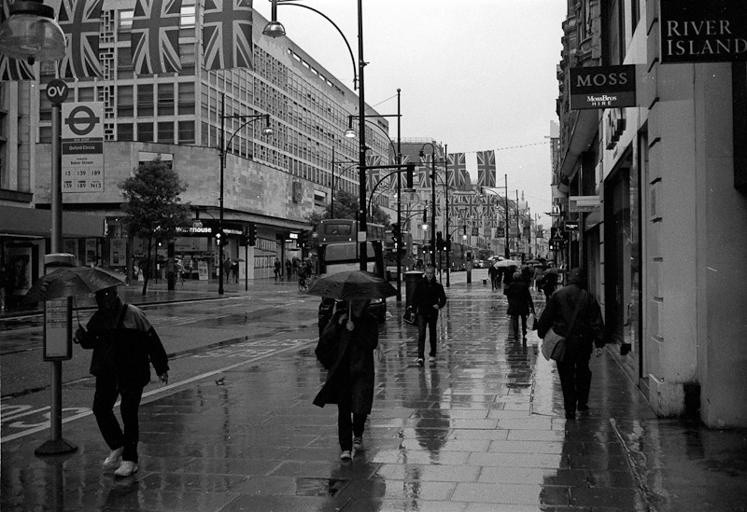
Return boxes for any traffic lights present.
[214,230,222,246]
[248,224,258,246]
[222,232,229,246]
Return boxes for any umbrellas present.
[233,258,246,263]
[24,267,127,344]
[306,271,399,330]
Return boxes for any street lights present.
[259,0,371,275]
[413,140,438,289]
[478,174,540,265]
[446,225,468,289]
[1,0,67,66]
[312,145,369,239]
[343,85,428,302]
[193,91,273,297]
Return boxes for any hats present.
[569,267,585,281]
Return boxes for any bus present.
[315,217,496,272]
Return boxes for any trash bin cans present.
[404,271,424,310]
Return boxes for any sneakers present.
[340,436,362,460]
[103,448,139,477]
[417,357,436,367]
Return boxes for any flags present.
[196,0,255,71]
[55,0,103,77]
[2,50,35,82]
[130,0,184,76]
[356,150,509,229]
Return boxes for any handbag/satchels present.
[541,326,567,362]
[402,308,418,326]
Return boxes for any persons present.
[406,265,447,368]
[77,286,170,477]
[316,298,379,460]
[506,273,535,339]
[537,268,606,419]
[223,258,232,283]
[232,261,240,284]
[273,255,315,289]
[488,255,567,293]
